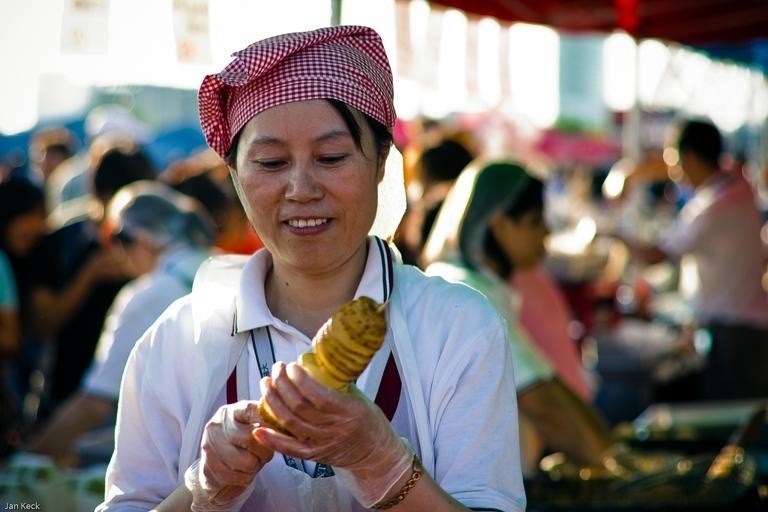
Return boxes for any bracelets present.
[367,454,422,510]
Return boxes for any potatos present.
[256,294,386,438]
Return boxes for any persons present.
[0,116,768,468]
[90,23,527,512]
